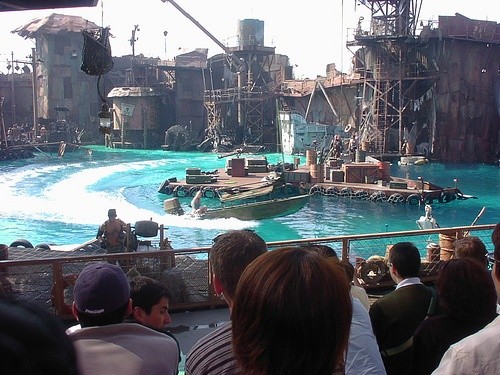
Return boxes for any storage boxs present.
[231,157,267,177]
[341,163,380,184]
[185,168,214,184]
[330,170,343,181]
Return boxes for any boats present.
[349,258,443,294]
[158,170,462,207]
[0,243,227,321]
[164,194,313,223]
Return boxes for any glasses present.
[485,252,500,264]
[212,229,256,247]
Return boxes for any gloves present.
[101,242,106,250]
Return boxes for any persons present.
[124,275,182,365]
[349,134,358,162]
[417,206,437,231]
[401,140,413,157]
[219,245,353,375]
[413,258,491,375]
[96,208,130,271]
[452,237,493,276]
[330,134,343,157]
[369,243,443,375]
[184,229,283,375]
[6,122,48,144]
[430,223,499,375]
[340,260,370,310]
[301,244,385,375]
[0,292,78,374]
[63,261,182,375]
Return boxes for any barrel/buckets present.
[439,231,469,260]
[310,164,324,183]
[356,151,365,162]
[426,244,440,261]
[362,142,369,153]
[306,150,317,166]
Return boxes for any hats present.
[72,264,131,315]
[107,209,117,216]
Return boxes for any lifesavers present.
[281,183,297,193]
[173,186,217,198]
[406,192,433,205]
[439,191,454,203]
